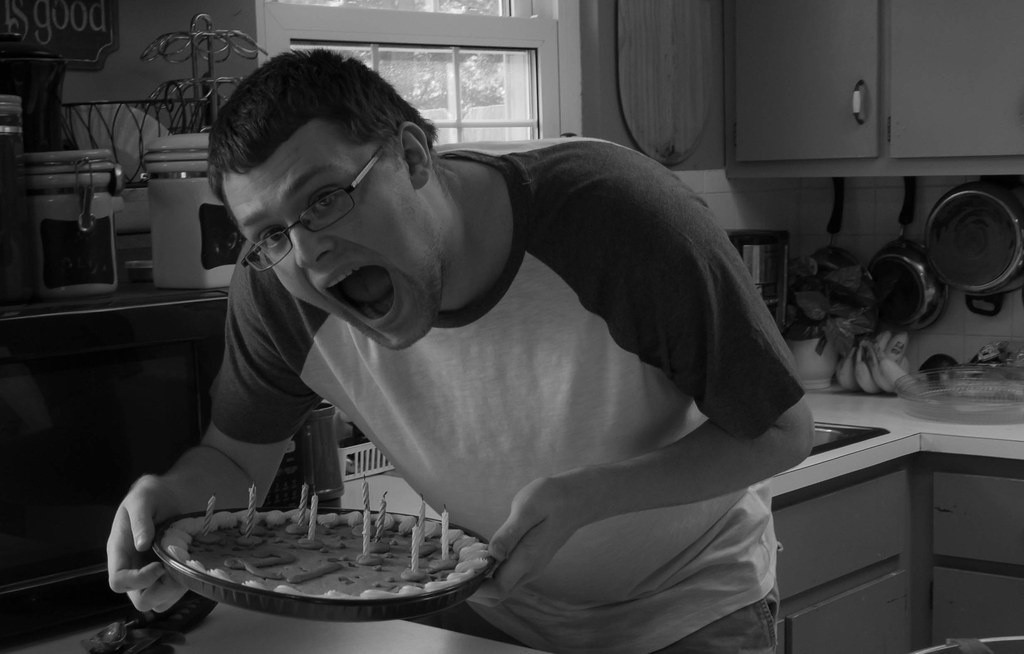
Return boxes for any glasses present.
[240,137,391,272]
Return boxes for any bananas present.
[835,329,913,393]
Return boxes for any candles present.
[411,516,422,575]
[301,479,309,527]
[308,484,317,539]
[363,504,372,556]
[362,474,370,512]
[442,504,449,561]
[374,490,389,543]
[244,481,258,538]
[201,487,218,537]
[419,492,426,547]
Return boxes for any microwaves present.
[0,290,229,642]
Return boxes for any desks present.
[0,602,551,654]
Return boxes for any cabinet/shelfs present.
[770,464,919,654]
[922,467,1024,649]
[721,0,1024,179]
[0,286,342,649]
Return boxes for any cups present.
[295,403,346,502]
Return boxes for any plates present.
[67,100,171,182]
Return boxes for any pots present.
[925,175,1024,316]
[801,176,853,285]
[866,177,948,332]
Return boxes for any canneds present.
[19,148,118,300]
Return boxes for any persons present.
[107,49,815,654]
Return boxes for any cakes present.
[158,509,491,599]
